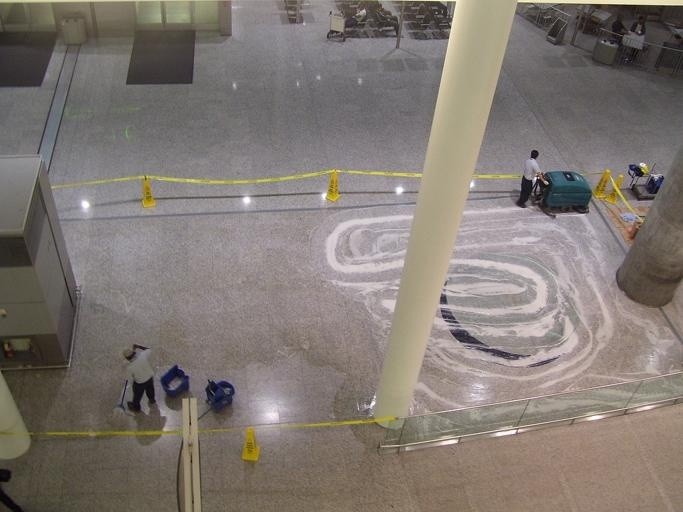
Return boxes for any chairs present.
[283,0,455,39]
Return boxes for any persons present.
[374,7,398,36]
[629,16,646,57]
[121,343,156,413]
[515,149,548,208]
[611,14,628,47]
[344,3,366,28]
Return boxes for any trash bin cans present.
[60,16,87,45]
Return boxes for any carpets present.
[126,30,195,84]
[0,30,59,88]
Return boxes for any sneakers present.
[127,402,140,412]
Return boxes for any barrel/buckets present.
[160,364,189,397]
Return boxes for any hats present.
[123,349,133,357]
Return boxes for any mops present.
[113,345,136,416]
